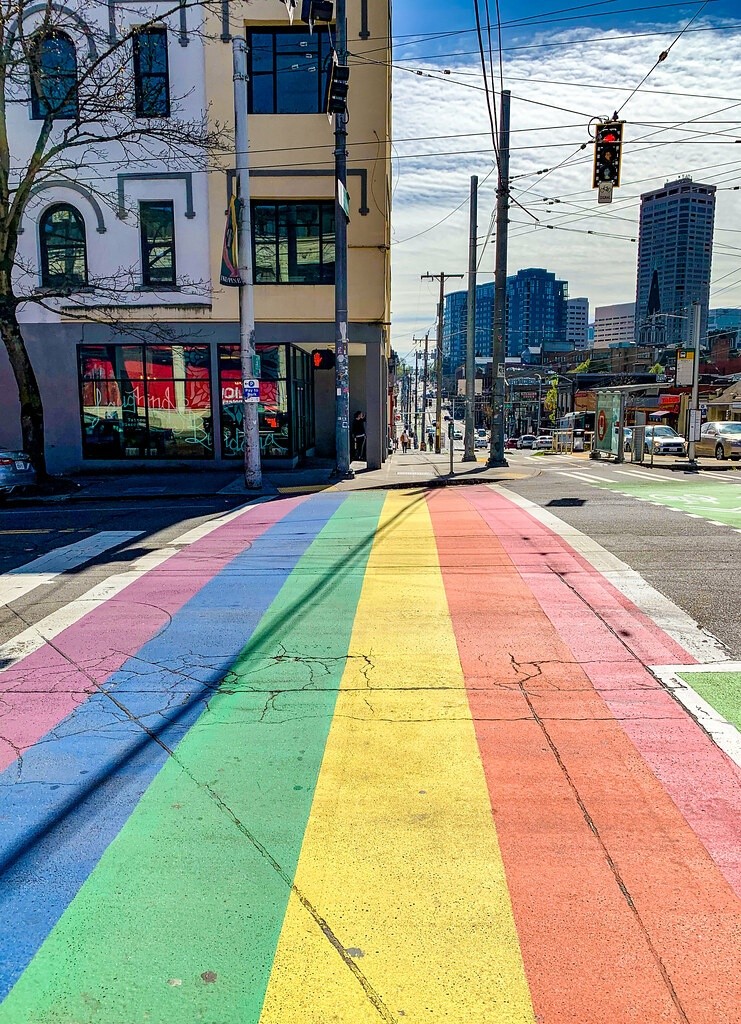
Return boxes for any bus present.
[552,410,597,451]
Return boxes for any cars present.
[453,430,463,440]
[426,420,436,437]
[444,415,454,421]
[685,420,741,460]
[475,439,487,448]
[505,438,519,450]
[83,412,117,454]
[625,424,687,456]
[0,445,39,508]
[591,425,632,450]
[105,417,176,448]
[531,436,553,451]
[478,429,487,437]
[202,402,290,437]
[516,434,537,450]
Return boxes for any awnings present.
[649,411,671,418]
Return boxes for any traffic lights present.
[302,0,332,34]
[593,121,623,187]
[324,49,350,122]
[311,348,335,370]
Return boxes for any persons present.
[401,429,409,453]
[428,434,434,452]
[352,410,367,461]
